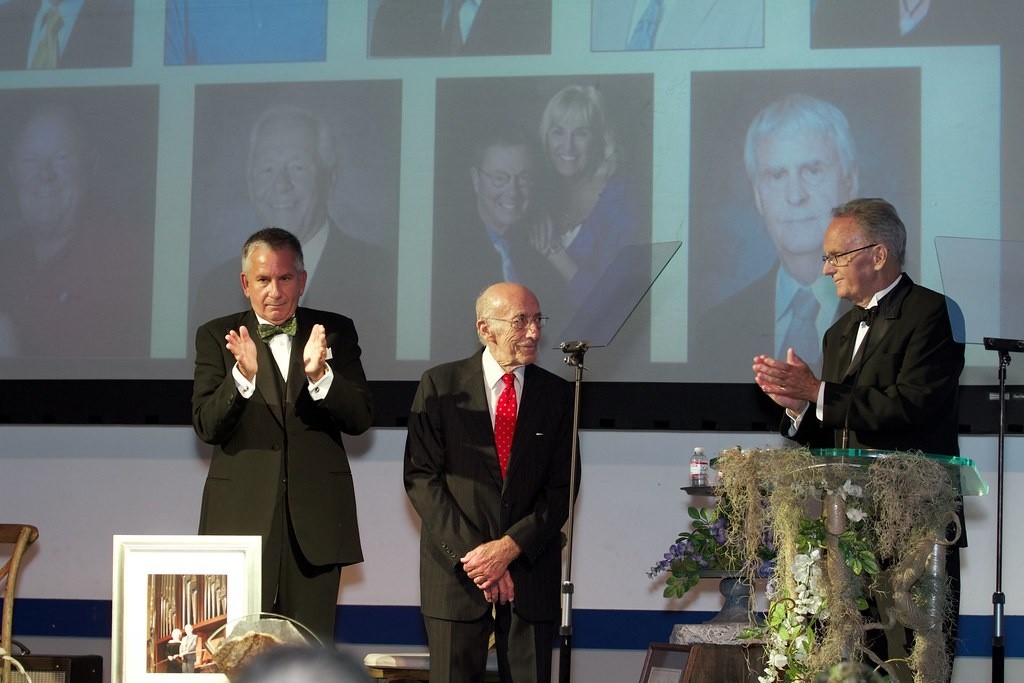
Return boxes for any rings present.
[779,380,787,387]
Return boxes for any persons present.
[812,0,997,48]
[0,0,133,71]
[370,0,552,54]
[167,629,182,672]
[699,95,857,384]
[0,98,150,358]
[440,84,646,355]
[591,1,763,49]
[180,624,198,672]
[230,645,374,682]
[164,0,327,64]
[403,282,581,683]
[191,226,374,646]
[197,109,385,366]
[752,199,967,682]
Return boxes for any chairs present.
[0,523,41,683]
[364,632,497,683]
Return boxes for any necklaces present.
[899,1,922,34]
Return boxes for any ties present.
[493,373,517,481]
[495,236,518,283]
[776,288,819,376]
[628,0,666,52]
[28,7,65,72]
[440,0,469,59]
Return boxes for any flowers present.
[646,504,779,600]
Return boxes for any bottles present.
[690,446,709,487]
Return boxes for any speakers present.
[0,655,103,683]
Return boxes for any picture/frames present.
[111,534,264,683]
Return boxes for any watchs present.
[546,242,563,259]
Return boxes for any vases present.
[695,569,771,622]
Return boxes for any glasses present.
[483,313,549,330]
[821,243,890,266]
[476,165,538,189]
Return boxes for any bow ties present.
[850,304,880,326]
[256,317,298,344]
